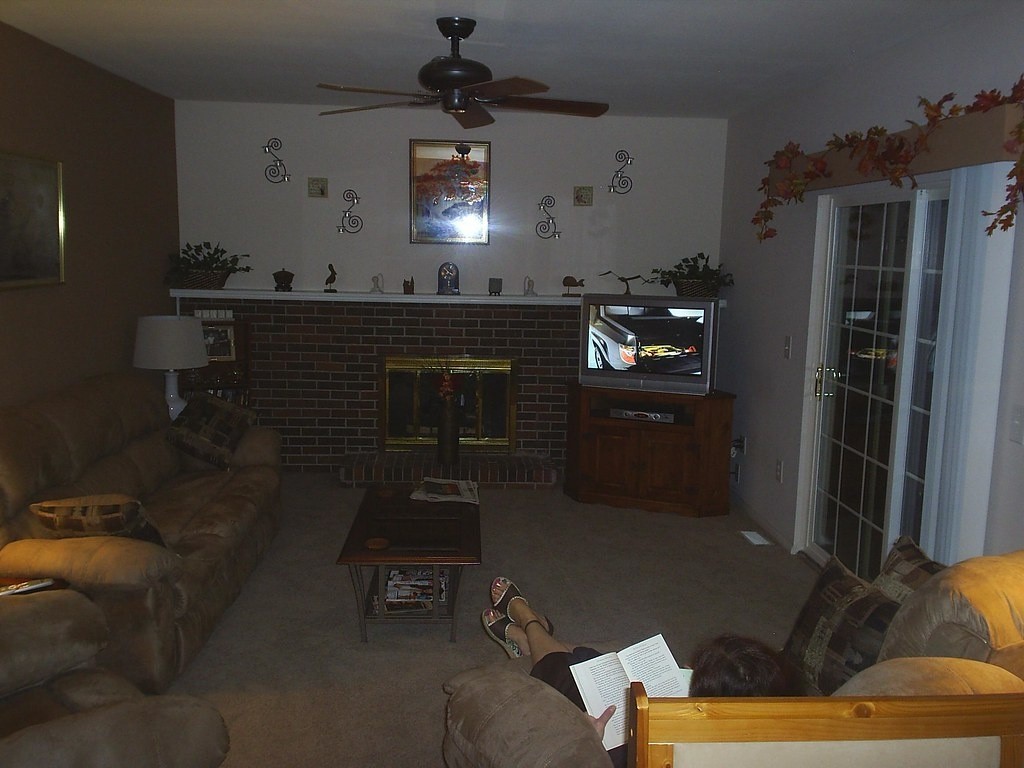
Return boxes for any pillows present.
[871,536,950,605]
[28,494,170,551]
[164,392,258,471]
[782,554,902,697]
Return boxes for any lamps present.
[132,316,209,421]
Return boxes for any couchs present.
[0,588,231,768]
[442,535,1024,768]
[0,373,282,695]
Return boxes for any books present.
[571,633,688,751]
[384,568,449,602]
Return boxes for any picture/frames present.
[409,138,491,246]
[0,148,66,290]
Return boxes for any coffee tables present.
[336,483,481,644]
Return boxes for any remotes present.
[0,576,55,597]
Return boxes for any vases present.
[438,405,459,465]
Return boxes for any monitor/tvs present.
[578,292,720,397]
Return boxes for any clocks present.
[437,262,460,295]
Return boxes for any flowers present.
[414,346,487,413]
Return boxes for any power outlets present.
[739,434,746,455]
[784,335,792,359]
[775,459,783,483]
[734,462,741,484]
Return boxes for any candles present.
[539,205,545,211]
[264,147,270,153]
[283,176,290,182]
[344,211,351,218]
[554,234,560,239]
[275,161,282,167]
[616,173,622,178]
[352,198,359,203]
[626,159,632,165]
[338,228,345,233]
[548,220,554,224]
[608,187,615,192]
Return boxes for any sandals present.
[481,577,554,660]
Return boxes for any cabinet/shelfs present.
[564,384,737,518]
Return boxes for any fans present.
[316,17,610,130]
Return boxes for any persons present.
[481,576,806,768]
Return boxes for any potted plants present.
[651,253,735,298]
[164,241,255,290]
